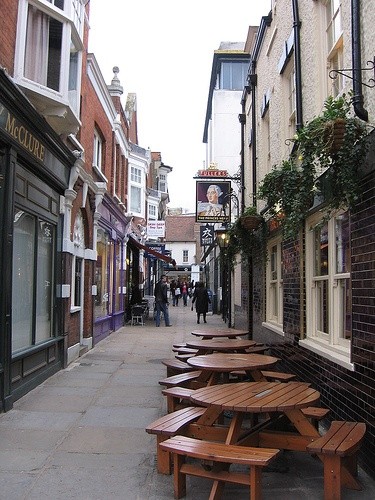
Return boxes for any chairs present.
[127,295,155,327]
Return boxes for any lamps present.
[214,219,231,250]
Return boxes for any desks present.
[190,382,363,500]
[191,329,250,340]
[186,339,257,356]
[187,353,278,387]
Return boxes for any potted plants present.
[219,89,371,272]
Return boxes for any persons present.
[192,283,212,324]
[153,275,172,327]
[197,185,229,216]
[169,279,197,307]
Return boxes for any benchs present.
[146,343,367,500]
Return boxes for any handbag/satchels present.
[175,288,181,295]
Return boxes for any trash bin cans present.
[143,299,149,318]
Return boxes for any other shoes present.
[166,324,172,327]
[156,324,159,327]
[204,321,206,323]
[197,321,200,324]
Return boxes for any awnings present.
[128,235,176,296]
